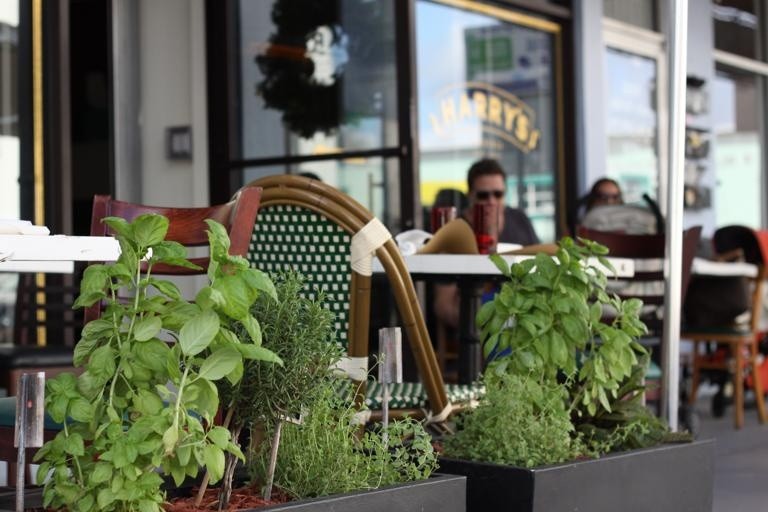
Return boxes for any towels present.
[0,218,50,234]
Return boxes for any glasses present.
[593,192,620,203]
[469,188,505,201]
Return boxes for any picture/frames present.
[163,125,192,159]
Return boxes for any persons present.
[583,175,627,219]
[432,156,544,346]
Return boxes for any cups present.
[473,203,499,254]
[430,208,457,237]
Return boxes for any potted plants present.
[27,216,467,512]
[407,237,716,512]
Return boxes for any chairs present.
[197,176,768,456]
[0,185,261,512]
